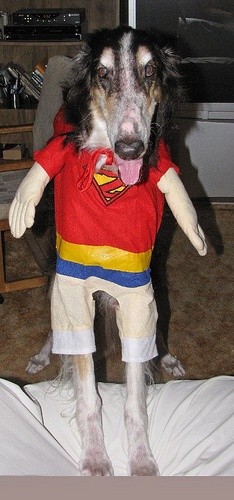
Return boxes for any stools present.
[0,168,49,294]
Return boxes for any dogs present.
[24,25,188,476]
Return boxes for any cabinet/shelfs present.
[0,40,87,172]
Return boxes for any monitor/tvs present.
[127,0,234,120]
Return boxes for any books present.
[0,143,26,160]
[0,63,46,108]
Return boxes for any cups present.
[0,11,11,41]
